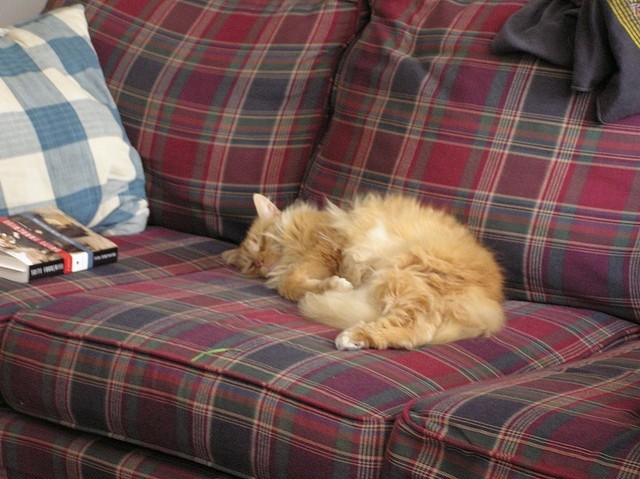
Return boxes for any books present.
[0,205,120,286]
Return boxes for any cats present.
[220,190,509,352]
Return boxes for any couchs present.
[0,0,640,478]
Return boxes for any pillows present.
[0,2,151,237]
